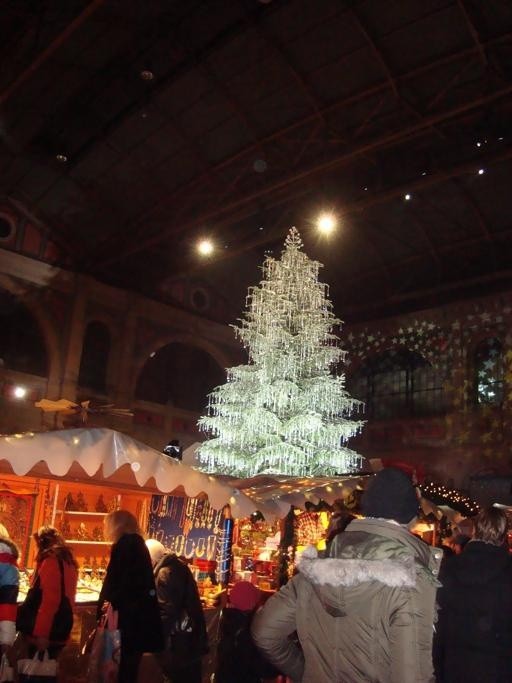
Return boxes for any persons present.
[214,581,280,683]
[14,525,79,683]
[436,506,512,683]
[248,464,436,683]
[145,538,208,682]
[96,510,159,682]
[321,512,512,570]
[0,522,20,683]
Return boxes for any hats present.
[360,465,418,524]
[230,581,259,611]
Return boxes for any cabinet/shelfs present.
[55,510,118,574]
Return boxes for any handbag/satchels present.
[16,588,73,641]
[79,628,121,683]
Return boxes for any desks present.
[16,572,104,658]
